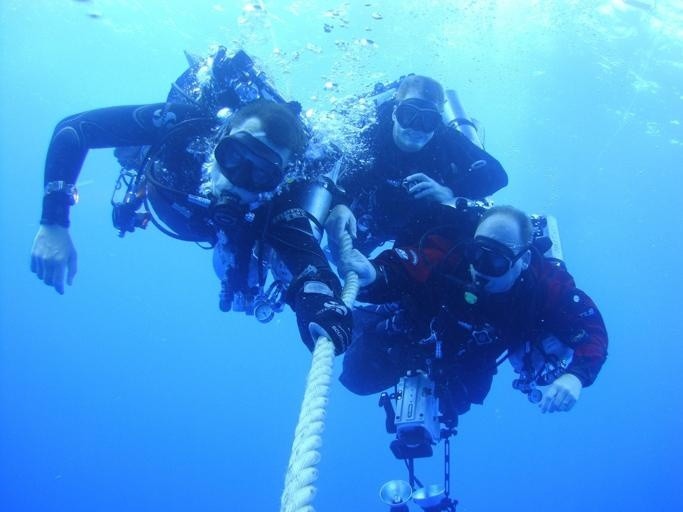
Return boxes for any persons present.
[325,71,510,269]
[336,198,609,413]
[28,48,355,355]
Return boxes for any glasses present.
[467,242,523,277]
[395,102,443,133]
[214,130,286,194]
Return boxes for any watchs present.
[43,181,78,205]
